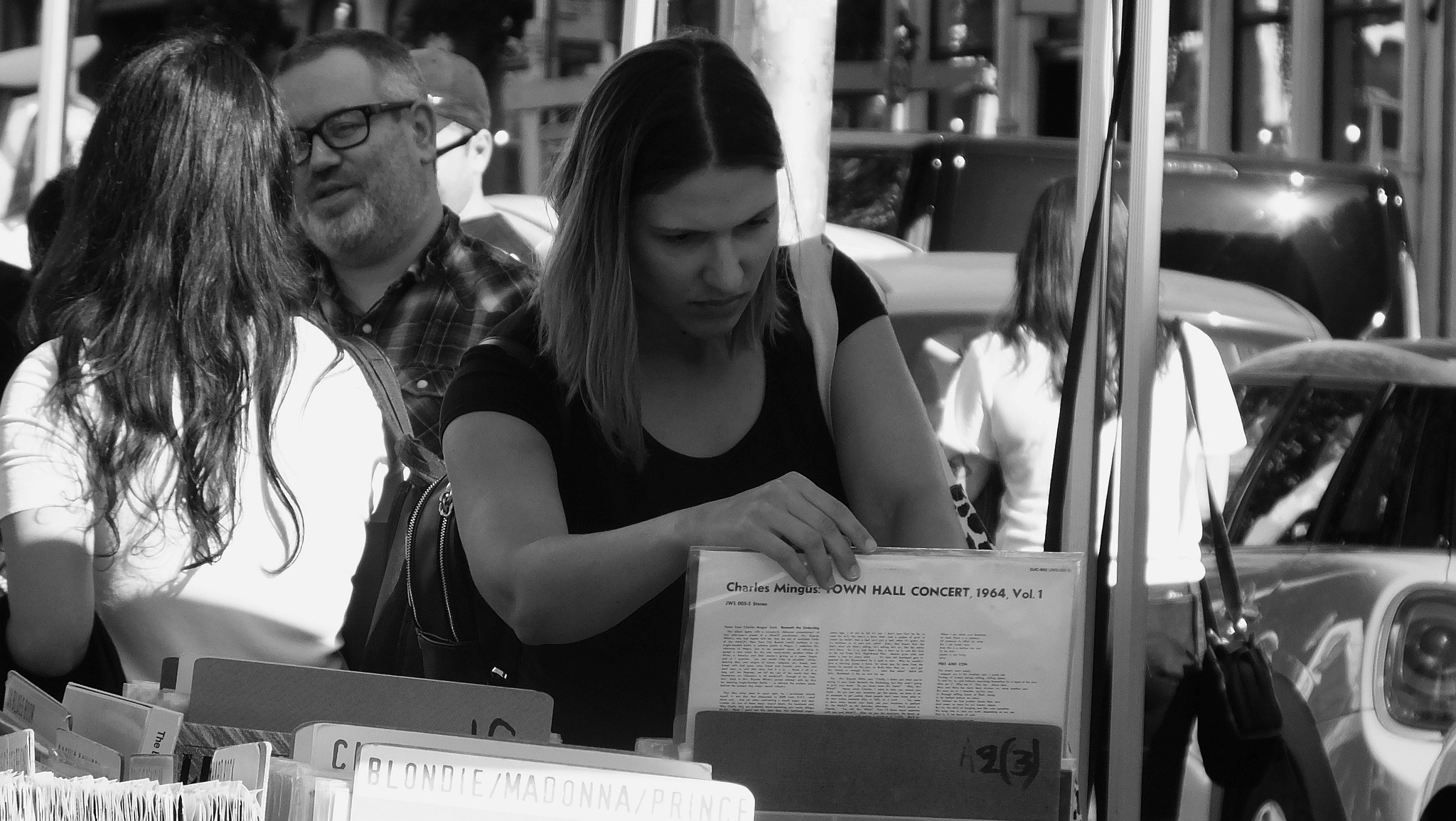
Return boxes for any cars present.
[821,223,1456,821]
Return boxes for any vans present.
[833,128,1424,343]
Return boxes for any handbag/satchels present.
[1198,633,1282,794]
[343,335,443,677]
[404,336,540,681]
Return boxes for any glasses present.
[288,99,414,165]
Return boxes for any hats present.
[410,49,492,131]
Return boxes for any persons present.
[0,32,419,689]
[439,27,970,750]
[933,174,1253,821]
[270,31,567,474]
